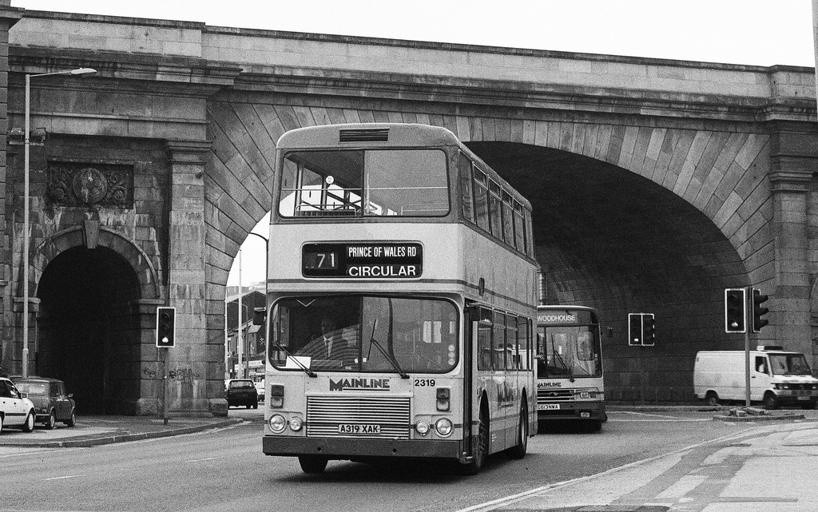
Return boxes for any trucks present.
[692,344,818,410]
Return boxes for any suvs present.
[8,374,77,429]
[224,378,260,410]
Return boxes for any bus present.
[261,119,540,478]
[538,304,614,431]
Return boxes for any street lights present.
[226,298,250,380]
[21,67,100,374]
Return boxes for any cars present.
[254,378,265,402]
[0,376,38,435]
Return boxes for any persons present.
[305,318,348,369]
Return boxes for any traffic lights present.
[156,307,176,347]
[628,313,643,346]
[642,313,655,346]
[725,289,747,333]
[751,287,769,333]
